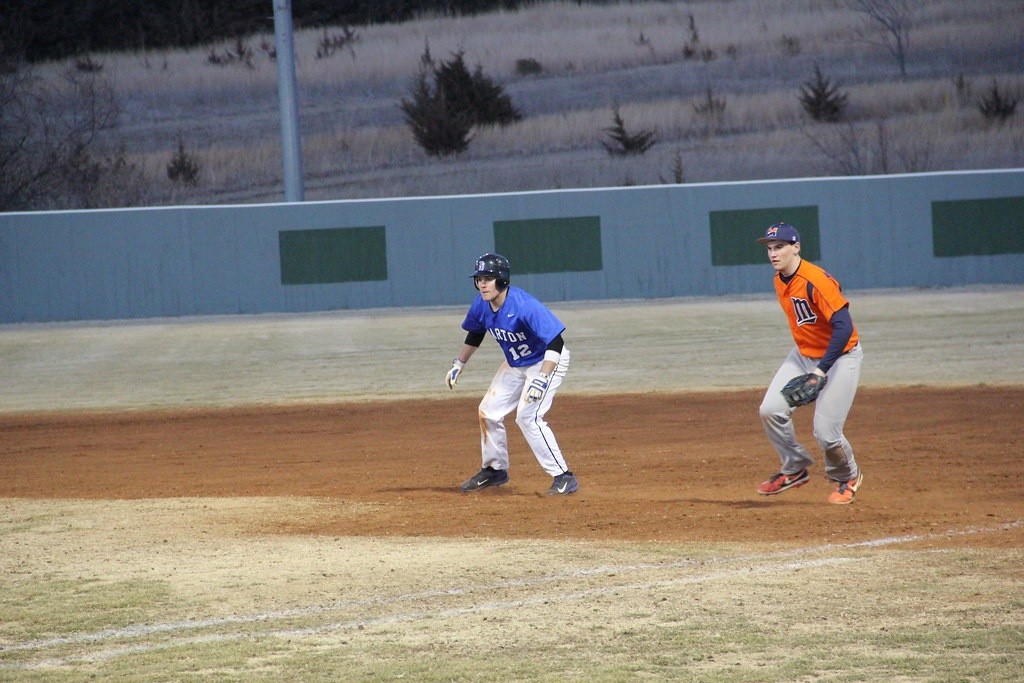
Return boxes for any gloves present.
[444,358,466,390]
[526,376,548,403]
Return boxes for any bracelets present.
[543,349,561,364]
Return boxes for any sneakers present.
[462,469,508,492]
[756,468,810,494]
[548,472,578,495]
[828,469,864,505]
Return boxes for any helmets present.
[469,252,510,289]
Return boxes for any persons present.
[445,253,579,496]
[756,222,862,504]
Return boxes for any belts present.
[841,342,858,356]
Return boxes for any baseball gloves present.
[780,373,829,407]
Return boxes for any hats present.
[757,222,800,243]
